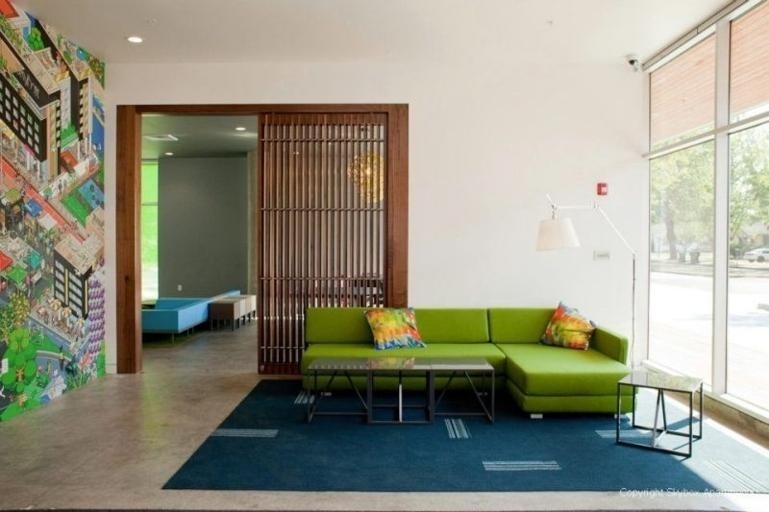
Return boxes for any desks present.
[616,367,705,457]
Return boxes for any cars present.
[743,248,769,263]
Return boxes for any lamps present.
[535,193,638,368]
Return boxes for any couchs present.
[300,303,638,420]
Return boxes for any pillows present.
[540,301,597,351]
[365,307,426,351]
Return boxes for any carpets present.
[160,379,769,492]
